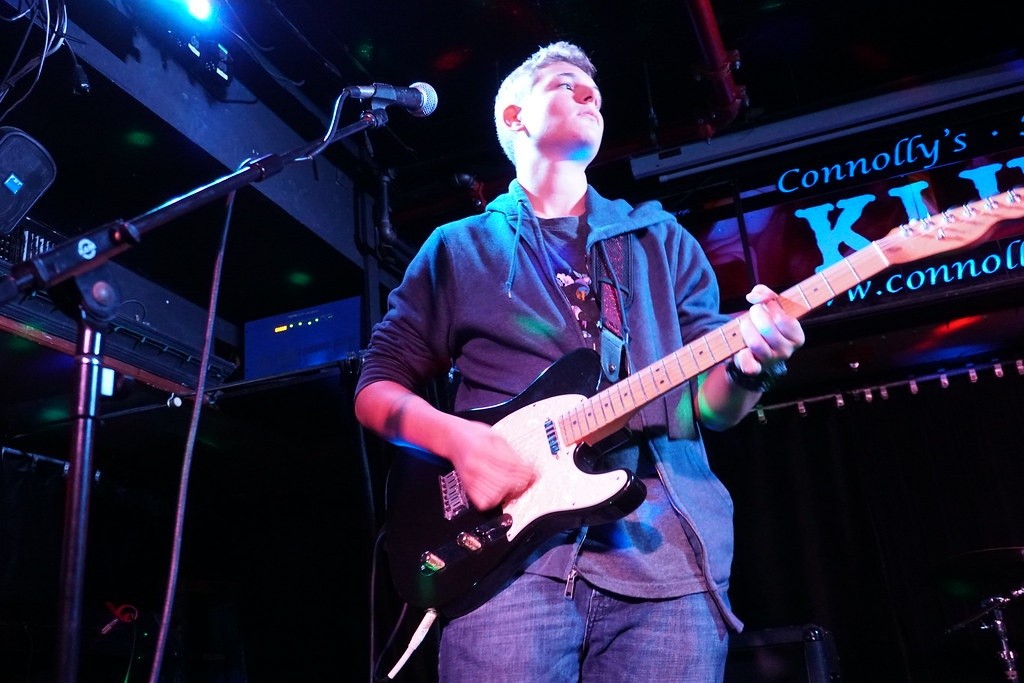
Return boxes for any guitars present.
[383,185,1024,622]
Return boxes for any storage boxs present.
[721,623,842,683]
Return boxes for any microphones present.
[345,81,439,117]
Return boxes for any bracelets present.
[724,355,787,393]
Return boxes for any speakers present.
[724,622,842,683]
[0,125,57,234]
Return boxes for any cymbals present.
[935,546,1024,597]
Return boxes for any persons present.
[353,42,805,683]
